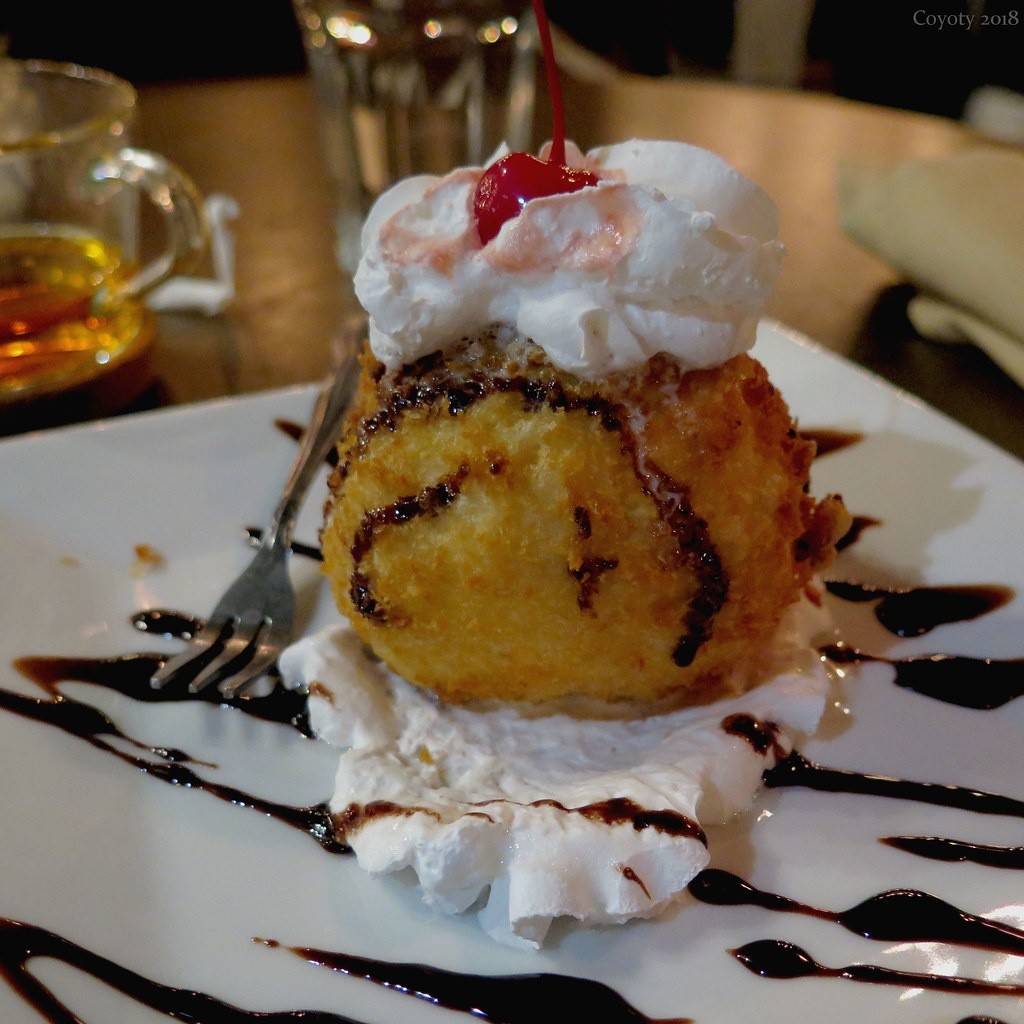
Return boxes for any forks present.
[150,311,370,700]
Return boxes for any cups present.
[1,57,210,402]
[297,0,539,303]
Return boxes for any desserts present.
[272,0,853,947]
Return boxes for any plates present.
[0,316,1024,1024]
